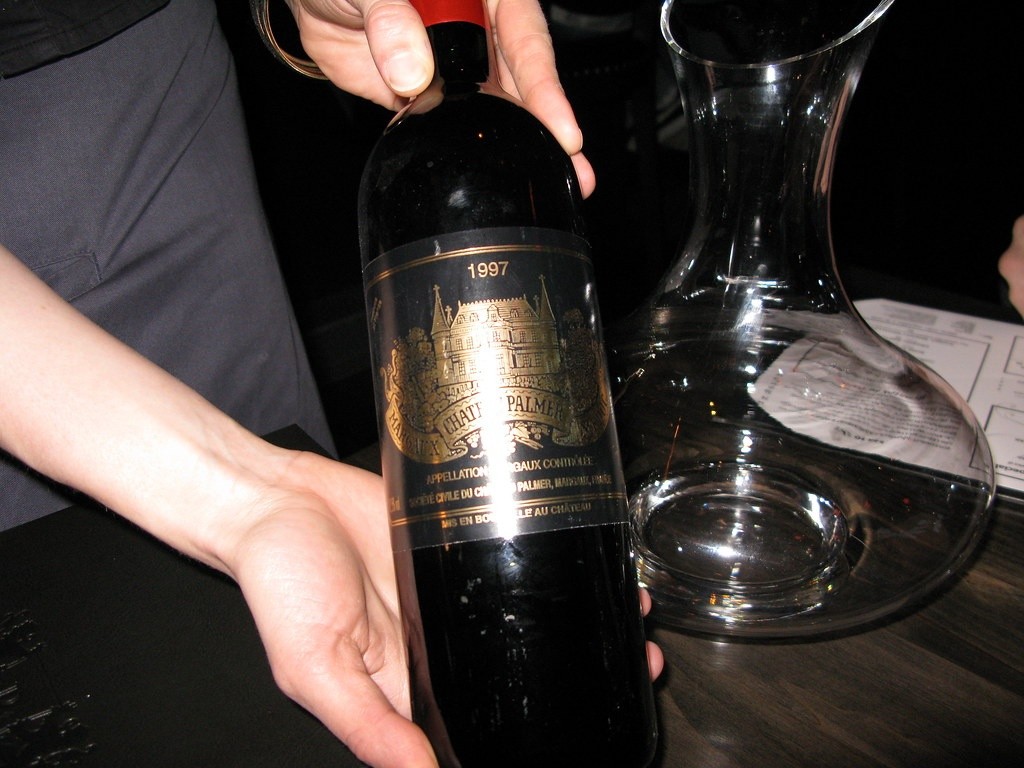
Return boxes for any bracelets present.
[249,0,331,81]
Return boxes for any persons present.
[0,0,666,768]
[998,215,1024,319]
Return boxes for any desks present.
[336,277,1024,768]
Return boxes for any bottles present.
[353,0,665,766]
[610,0,998,640]
